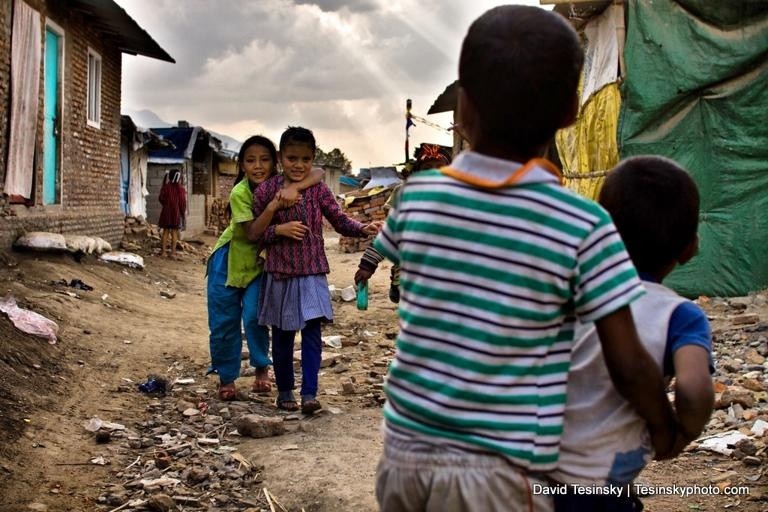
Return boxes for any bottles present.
[356,276,368,309]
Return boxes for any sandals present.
[301,395,321,413]
[254,369,272,392]
[277,390,299,410]
[220,382,236,399]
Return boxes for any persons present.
[203,135,327,402]
[158,169,187,257]
[546,155,717,512]
[373,5,679,512]
[354,145,451,303]
[253,126,387,414]
